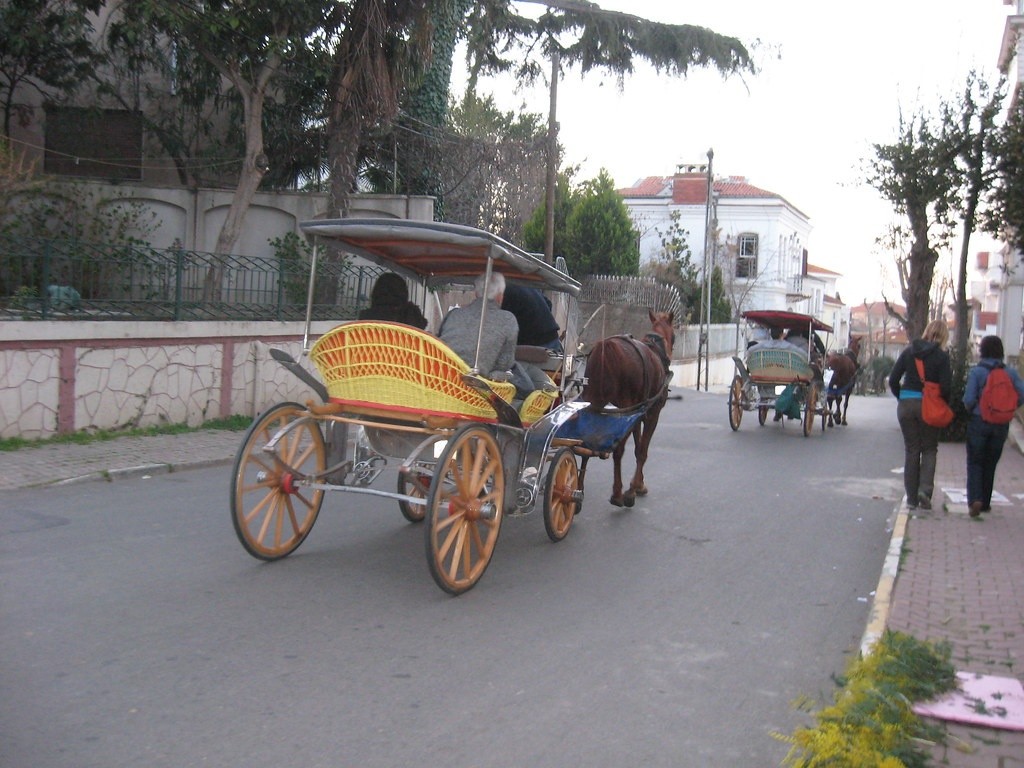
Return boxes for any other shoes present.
[907,499,916,511]
[970,502,982,517]
[918,491,932,509]
[983,502,991,512]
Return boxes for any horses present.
[827,335,862,428]
[574,309,676,515]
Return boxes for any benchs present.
[514,345,573,379]
[745,349,815,383]
[509,361,560,428]
[313,320,515,419]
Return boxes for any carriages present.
[727,309,862,438]
[229,218,675,598]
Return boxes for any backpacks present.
[978,362,1018,424]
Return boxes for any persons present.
[961,336,1024,517]
[889,320,953,510]
[500,282,565,352]
[747,325,828,408]
[357,272,430,329]
[437,272,525,412]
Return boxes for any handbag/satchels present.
[915,357,954,428]
